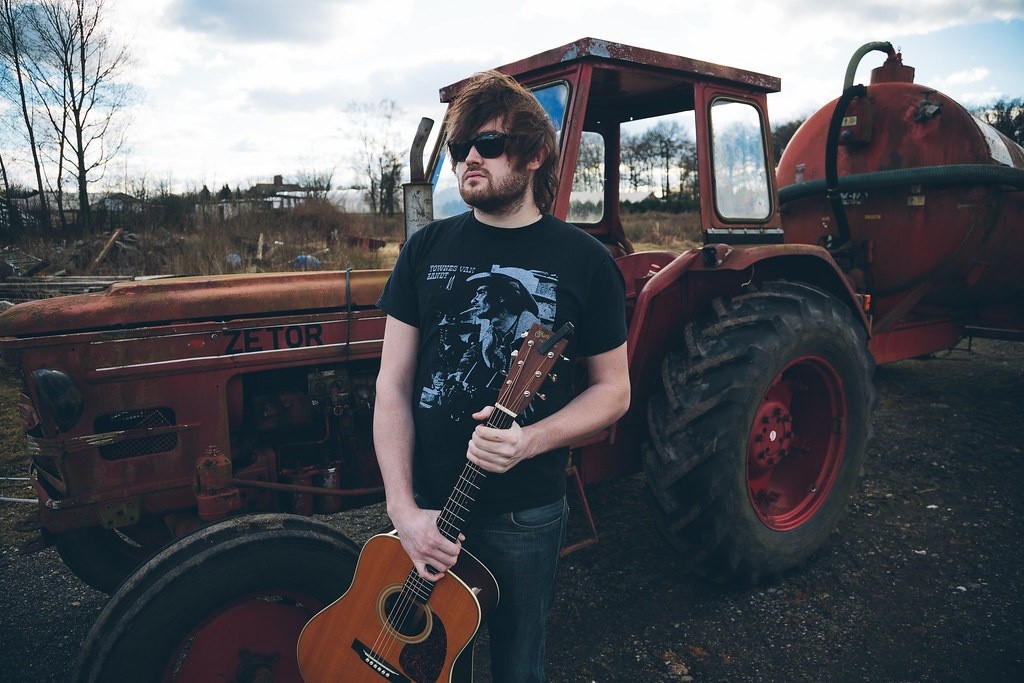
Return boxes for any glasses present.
[447,131,515,163]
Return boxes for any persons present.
[372,67,631,683]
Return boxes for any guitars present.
[295,321,577,683]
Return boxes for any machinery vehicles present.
[0,36,1023,682]
